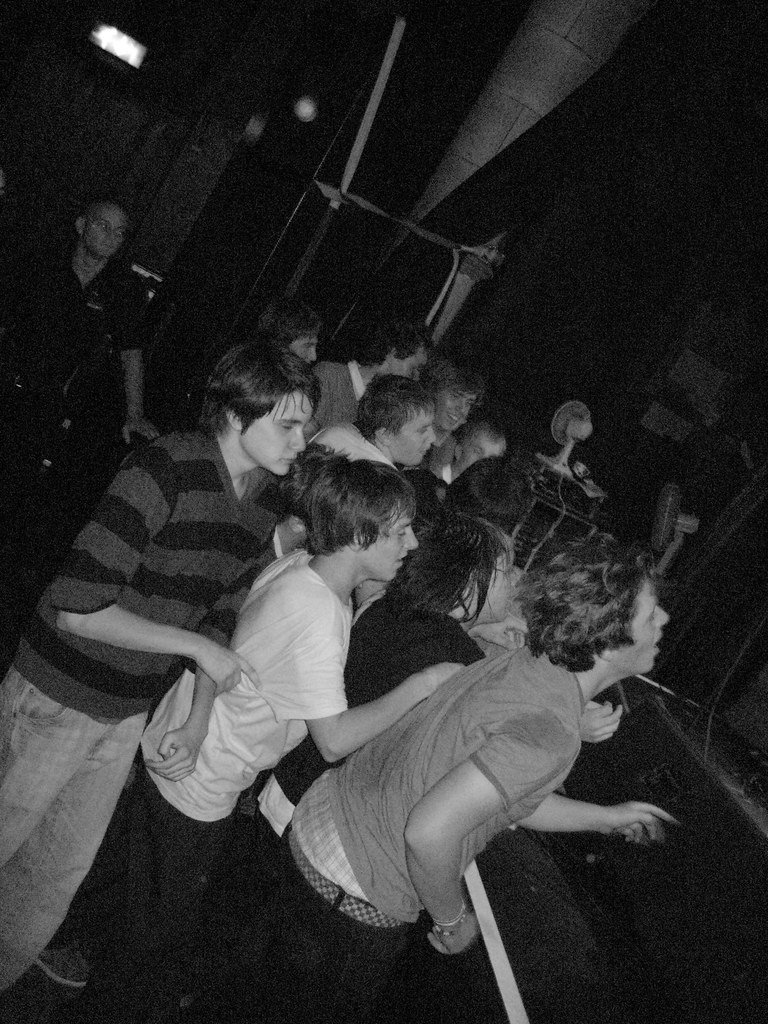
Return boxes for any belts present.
[287,829,408,928]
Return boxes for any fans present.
[651,480,702,576]
[535,399,595,481]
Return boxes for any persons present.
[0,164,623,858]
[129,456,419,950]
[290,534,684,953]
[0,340,318,995]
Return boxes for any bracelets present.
[433,897,467,937]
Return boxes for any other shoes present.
[35,947,92,988]
